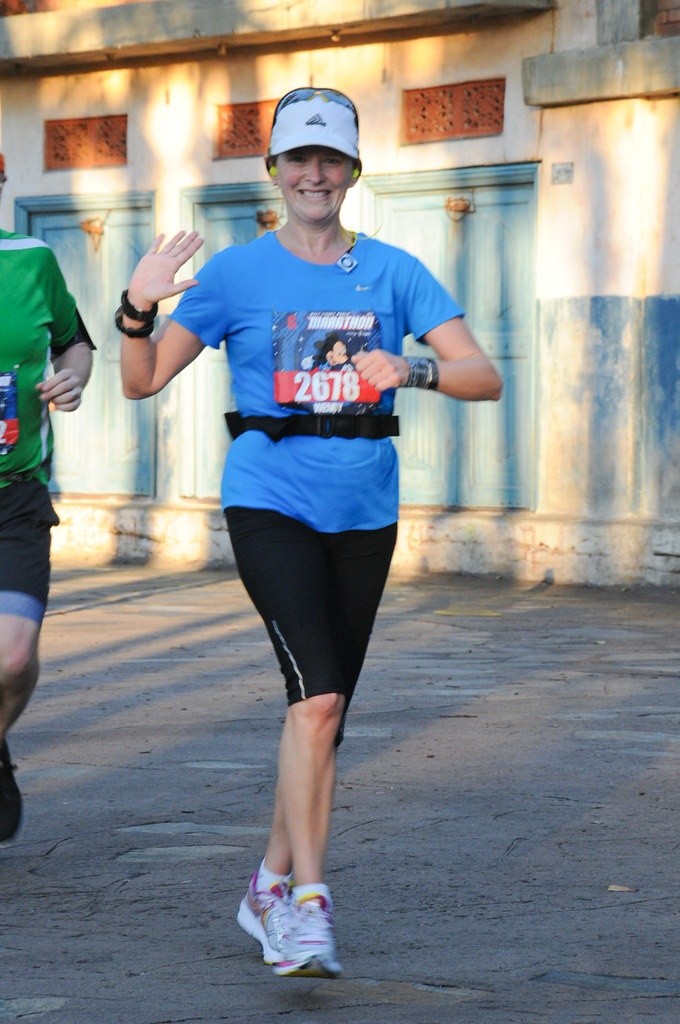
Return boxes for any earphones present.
[351,168,360,179]
[270,165,278,177]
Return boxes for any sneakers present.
[0,739,23,848]
[234,867,344,978]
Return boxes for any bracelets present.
[404,356,430,390]
[115,306,155,338]
[426,359,438,389]
[121,289,158,322]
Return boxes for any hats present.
[268,93,360,159]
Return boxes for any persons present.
[0,154,98,844]
[120,88,507,980]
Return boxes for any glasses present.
[273,87,359,128]
